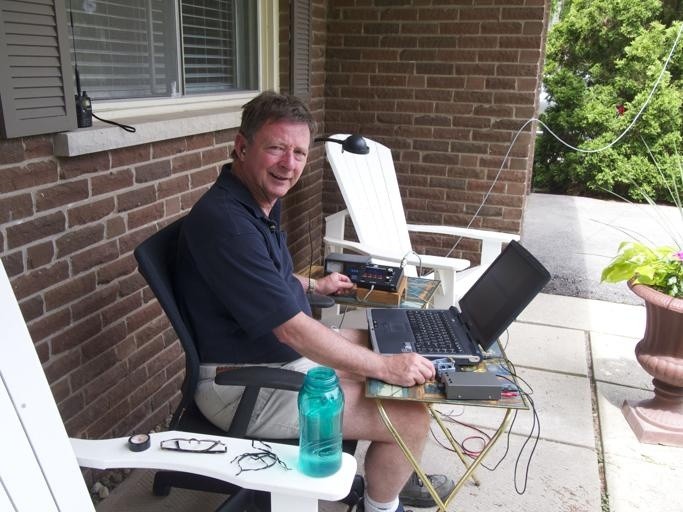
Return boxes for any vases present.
[623,277,683,447]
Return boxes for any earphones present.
[242,147,246,154]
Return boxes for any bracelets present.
[307,278,316,294]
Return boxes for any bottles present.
[296,367,344,478]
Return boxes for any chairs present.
[0,260,358,512]
[134,213,365,512]
[322,133,521,310]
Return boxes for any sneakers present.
[399,472,455,508]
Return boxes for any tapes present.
[128,434,150,452]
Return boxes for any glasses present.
[160,437,227,453]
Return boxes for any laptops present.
[365,239,551,366]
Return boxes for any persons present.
[172,88,436,512]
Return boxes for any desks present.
[297,264,441,321]
[364,337,530,511]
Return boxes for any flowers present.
[598,133,683,298]
